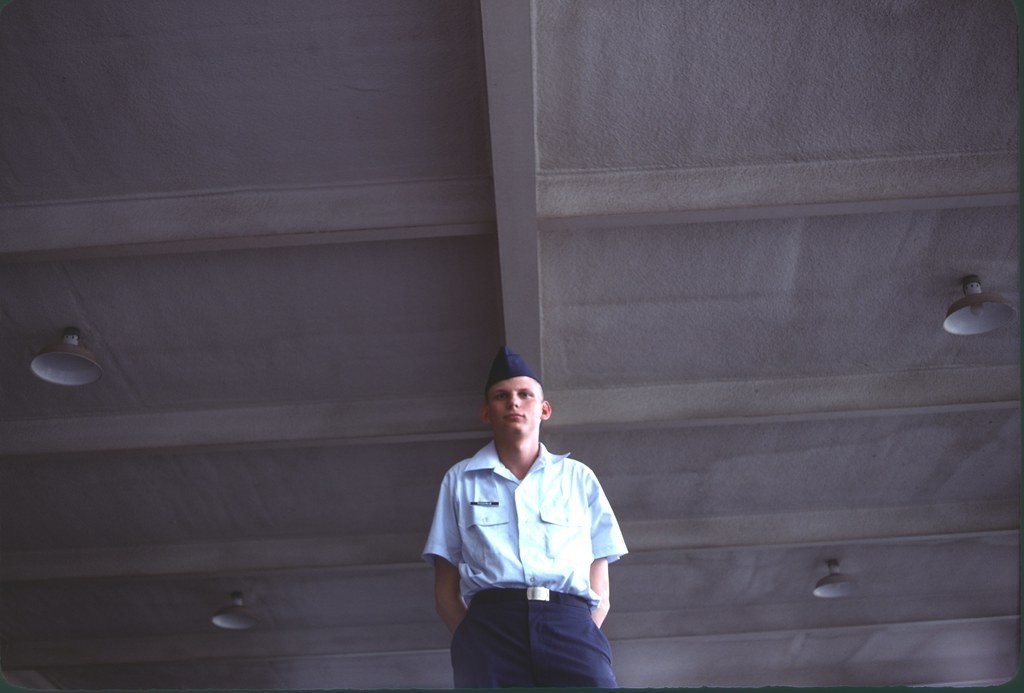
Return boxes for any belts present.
[482,586,589,610]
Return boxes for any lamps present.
[31,328,103,388]
[813,558,862,599]
[942,274,1013,338]
[212,590,264,630]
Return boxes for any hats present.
[484,344,544,401]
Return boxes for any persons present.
[422,346,628,693]
